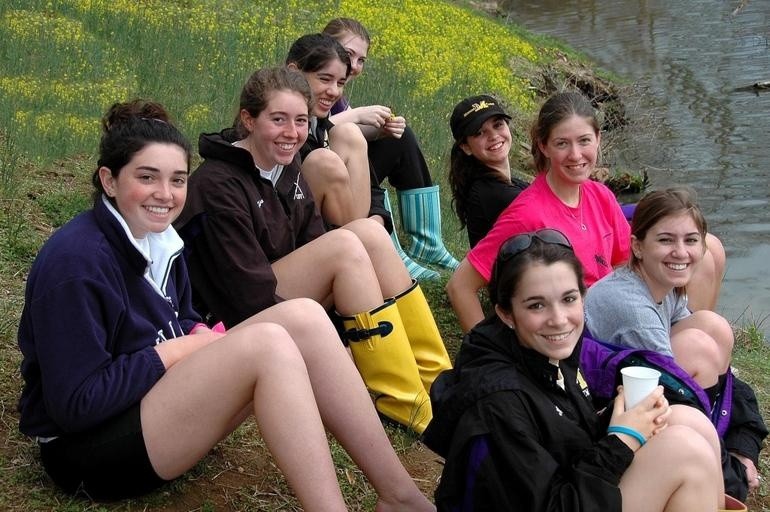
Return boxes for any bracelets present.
[604,425,648,448]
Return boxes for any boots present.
[334,279,454,435]
[382,189,440,280]
[396,185,461,269]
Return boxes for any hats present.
[450,94,512,145]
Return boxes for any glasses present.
[494,228,575,288]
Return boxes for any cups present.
[620,366,661,412]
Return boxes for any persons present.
[582,185,763,493]
[177,72,452,437]
[431,230,728,512]
[16,100,432,511]
[443,94,724,353]
[287,39,394,233]
[446,101,636,262]
[319,18,458,283]
